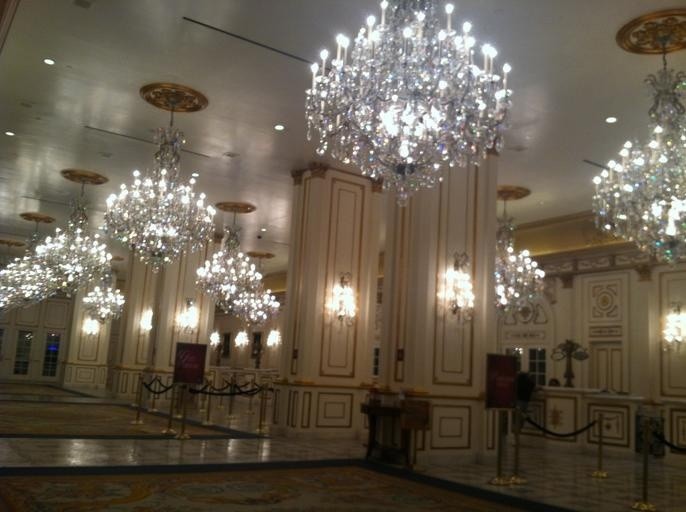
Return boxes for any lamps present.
[589,8,686,262]
[493,185,547,317]
[1,165,126,326]
[437,249,475,321]
[324,272,358,326]
[306,0,513,207]
[194,195,282,326]
[550,339,589,388]
[662,304,686,344]
[102,82,218,276]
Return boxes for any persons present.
[549,379,559,386]
[515,368,544,433]
